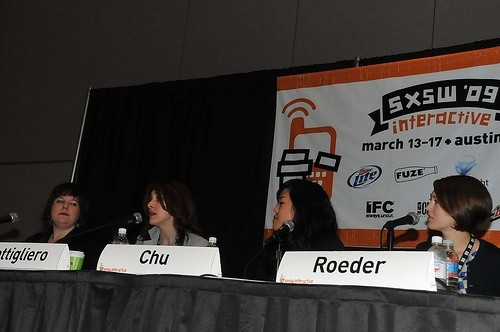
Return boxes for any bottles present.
[113,228,129,245]
[207,237,217,247]
[428,236,448,291]
[443,239,459,292]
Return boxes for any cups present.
[69,250,85,270]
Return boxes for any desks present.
[0,271,500,332]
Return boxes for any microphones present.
[0,213,19,224]
[262,220,295,246]
[110,213,143,227]
[383,212,420,229]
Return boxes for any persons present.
[415,175,500,297]
[246,179,345,282]
[128,178,209,246]
[24,182,105,270]
